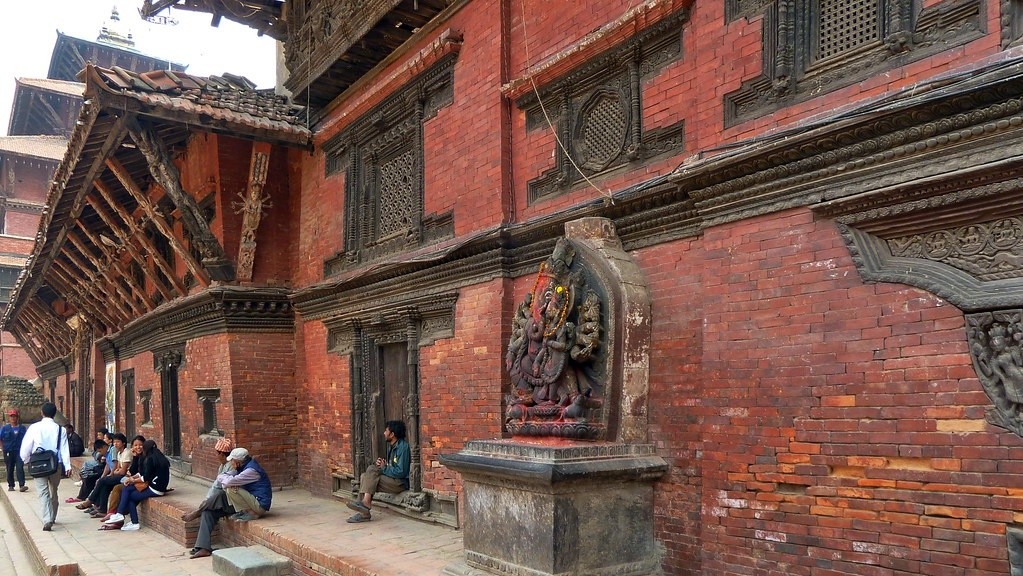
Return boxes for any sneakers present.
[120,521,141,531]
[105,513,125,524]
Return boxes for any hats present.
[9,410,17,416]
[215,438,234,452]
[226,448,249,462]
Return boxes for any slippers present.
[66,498,81,503]
[84,506,97,513]
[91,512,105,518]
[190,548,198,553]
[91,508,102,513]
[346,512,371,523]
[345,501,369,514]
[76,499,94,509]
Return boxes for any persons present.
[180,438,236,559]
[65,427,170,531]
[345,419,411,523]
[230,180,274,242]
[20,402,73,531]
[59,424,84,479]
[216,447,272,523]
[0,409,29,492]
[504,235,602,407]
[26,190,166,349]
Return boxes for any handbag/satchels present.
[28,446,58,478]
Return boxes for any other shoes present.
[231,511,260,522]
[20,485,28,492]
[101,512,115,521]
[9,486,14,491]
[42,522,50,530]
[99,525,117,531]
